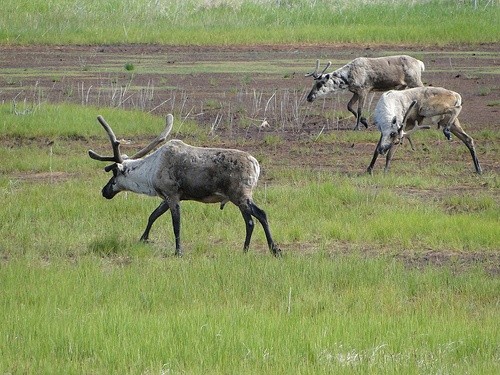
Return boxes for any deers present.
[304,55,426,130]
[88,112,283,259]
[367,87,479,176]
